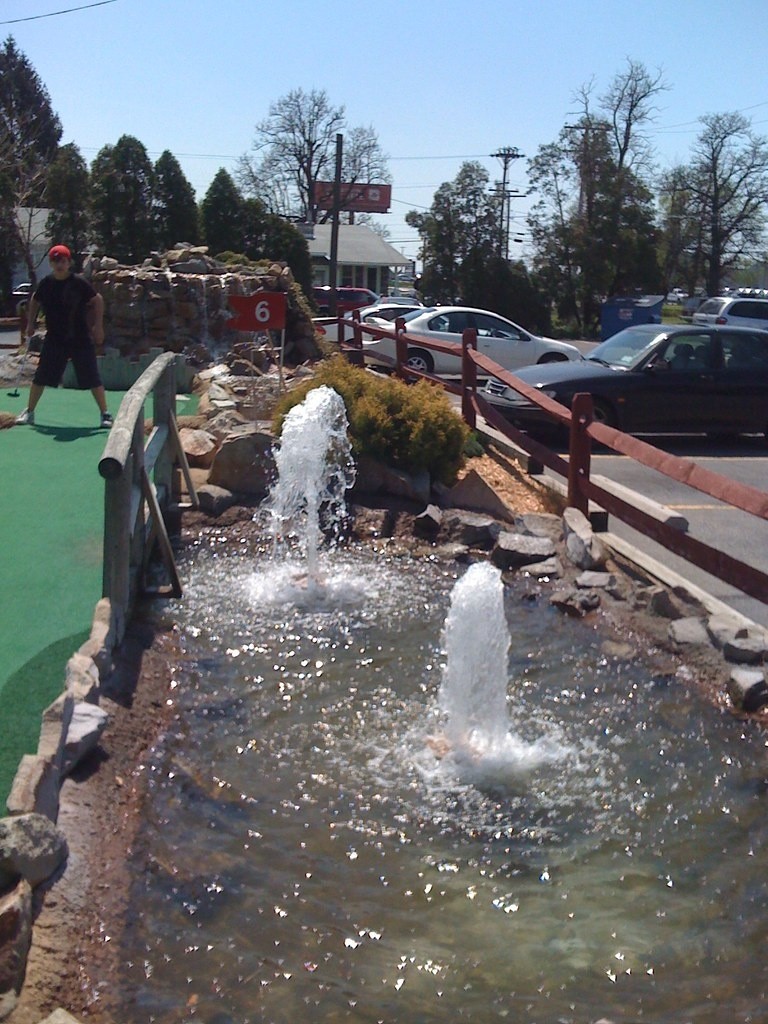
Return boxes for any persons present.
[17,245,114,428]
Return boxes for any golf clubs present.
[6,335,33,398]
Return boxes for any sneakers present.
[100,412,113,427]
[13,407,34,424]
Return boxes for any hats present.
[50,245,70,261]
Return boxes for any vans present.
[312,287,379,317]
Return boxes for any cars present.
[11,282,32,300]
[481,323,768,449]
[666,288,768,330]
[311,296,584,376]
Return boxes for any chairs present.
[671,344,694,365]
[689,346,708,368]
